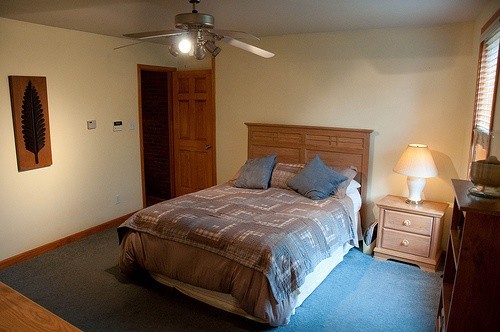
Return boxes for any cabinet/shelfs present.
[434,178,500,332]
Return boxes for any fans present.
[113,0,277,60]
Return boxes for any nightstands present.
[372,193,449,273]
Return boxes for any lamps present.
[166,32,223,60]
[388,141,437,206]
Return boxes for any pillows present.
[287,154,350,200]
[234,152,278,189]
[272,161,304,192]
[332,164,358,196]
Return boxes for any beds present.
[114,122,374,326]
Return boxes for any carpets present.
[0,221,450,332]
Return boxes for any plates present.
[468,186,500,198]
